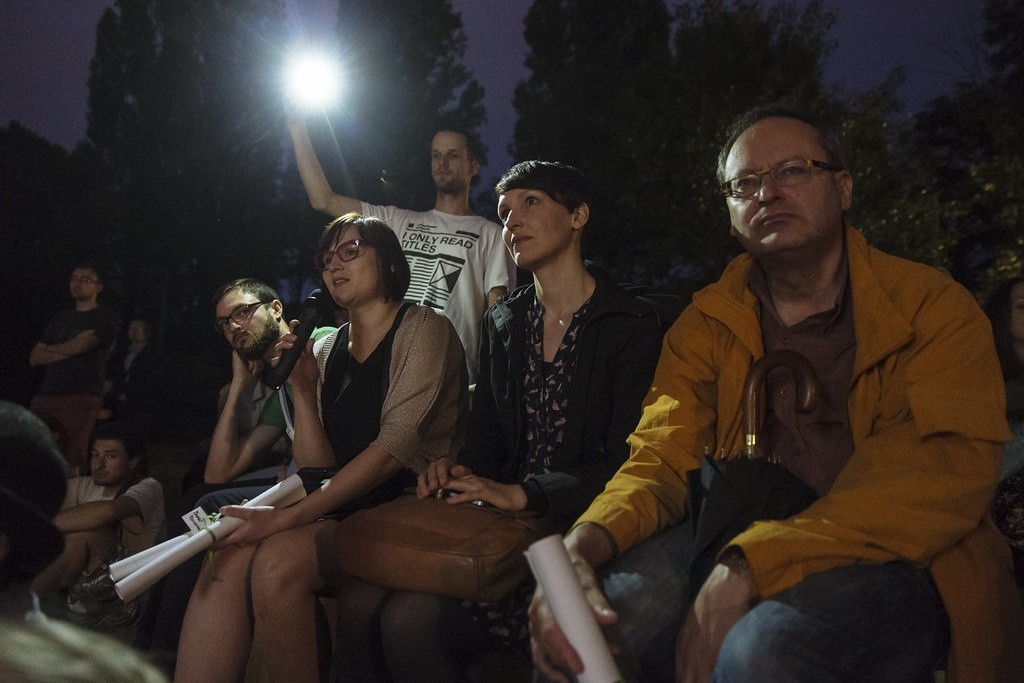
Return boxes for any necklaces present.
[558,307,580,324]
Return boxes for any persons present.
[985,278,1024,578]
[129,274,339,683]
[332,160,668,683]
[528,100,1024,683]
[172,212,471,683]
[89,314,166,444]
[190,383,291,480]
[32,421,166,632]
[0,402,170,683]
[29,263,123,465]
[289,117,516,375]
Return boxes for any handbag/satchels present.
[296,467,418,521]
[334,485,552,603]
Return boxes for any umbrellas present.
[686,348,819,603]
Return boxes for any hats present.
[0,400,70,562]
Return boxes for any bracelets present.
[729,552,758,599]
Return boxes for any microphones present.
[274,289,333,381]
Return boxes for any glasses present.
[214,297,277,334]
[719,158,839,199]
[128,327,151,335]
[70,275,101,286]
[312,239,389,271]
